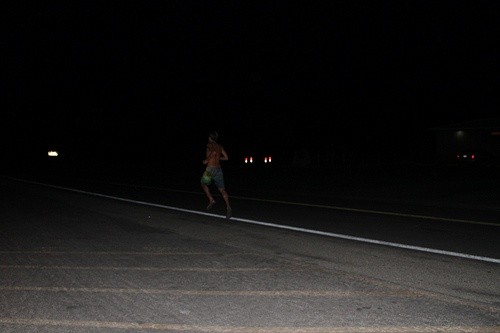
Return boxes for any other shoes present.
[207,200,216,210]
[226,206,232,218]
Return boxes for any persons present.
[200,129,231,217]
